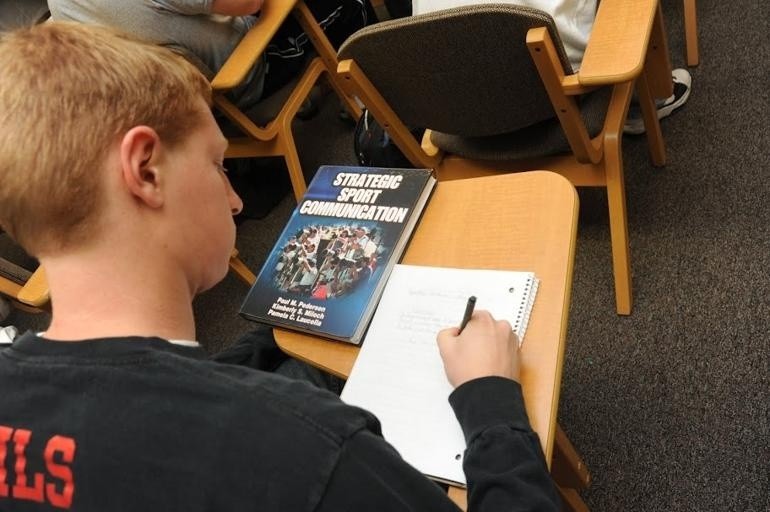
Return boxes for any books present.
[237,156,438,345]
[332,260,539,493]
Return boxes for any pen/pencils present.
[459,296,477,331]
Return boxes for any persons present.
[0,19,567,512]
[44,2,384,113]
[411,1,694,164]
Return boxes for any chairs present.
[201,1,364,210]
[335,0,666,317]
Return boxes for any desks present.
[271,172,583,453]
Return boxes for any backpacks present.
[352,106,419,170]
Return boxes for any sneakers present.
[622,66,694,135]
